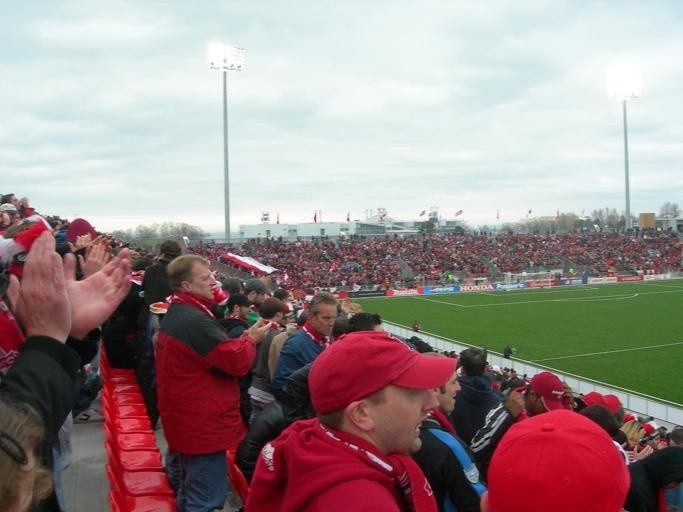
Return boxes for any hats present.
[227,293,252,306]
[160,240,181,255]
[624,415,634,422]
[308,331,457,415]
[580,392,604,407]
[529,372,563,411]
[644,424,655,435]
[243,278,270,294]
[487,410,630,512]
[1,203,22,214]
[603,395,622,413]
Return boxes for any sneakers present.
[72,409,104,424]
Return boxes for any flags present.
[314,213,316,222]
[420,210,425,216]
[455,210,462,216]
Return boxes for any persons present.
[152,253,274,512]
[1,192,213,511]
[243,330,459,512]
[214,231,683,512]
[0,229,134,512]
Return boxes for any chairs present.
[96,341,262,512]
